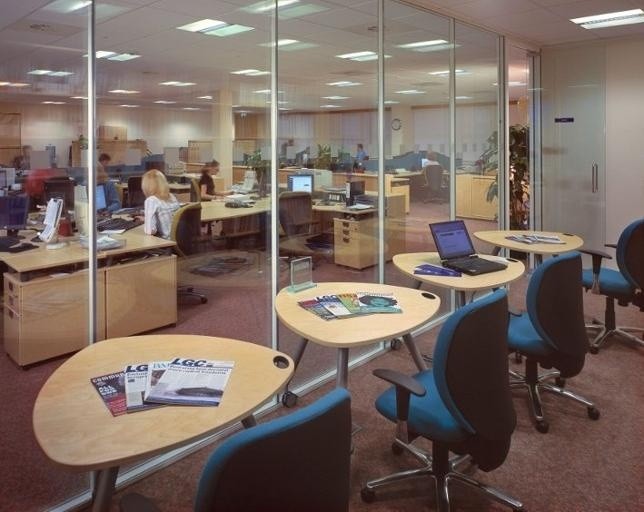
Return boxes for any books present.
[91,370,127,417]
[145,357,235,407]
[296,288,405,323]
[124,364,169,414]
[144,361,172,404]
[504,231,565,247]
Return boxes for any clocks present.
[390,118,401,130]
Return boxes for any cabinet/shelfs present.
[69,139,145,168]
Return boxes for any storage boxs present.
[97,125,126,141]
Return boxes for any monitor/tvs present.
[97,183,108,222]
[287,174,313,194]
[0,171,7,190]
[0,196,30,238]
[0,168,15,185]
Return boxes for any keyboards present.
[98,218,126,230]
[118,218,144,232]
[0,239,19,247]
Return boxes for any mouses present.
[133,217,139,222]
[22,243,33,249]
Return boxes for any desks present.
[272,278,443,416]
[472,227,584,270]
[31,333,296,511]
[389,246,527,313]
[3,166,178,370]
[178,163,451,270]
[455,165,498,222]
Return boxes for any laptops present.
[428,220,508,276]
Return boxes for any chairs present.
[580,216,643,355]
[116,386,354,509]
[505,249,601,435]
[359,287,526,511]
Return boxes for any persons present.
[133,169,182,257]
[199,160,236,201]
[95,153,111,179]
[295,146,309,159]
[421,149,441,186]
[282,140,294,156]
[80,161,121,218]
[12,146,32,170]
[353,143,365,160]
